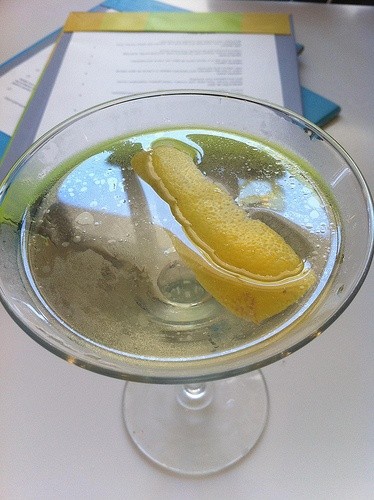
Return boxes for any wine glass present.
[0,86,374,478]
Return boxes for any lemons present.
[129,146,317,321]
